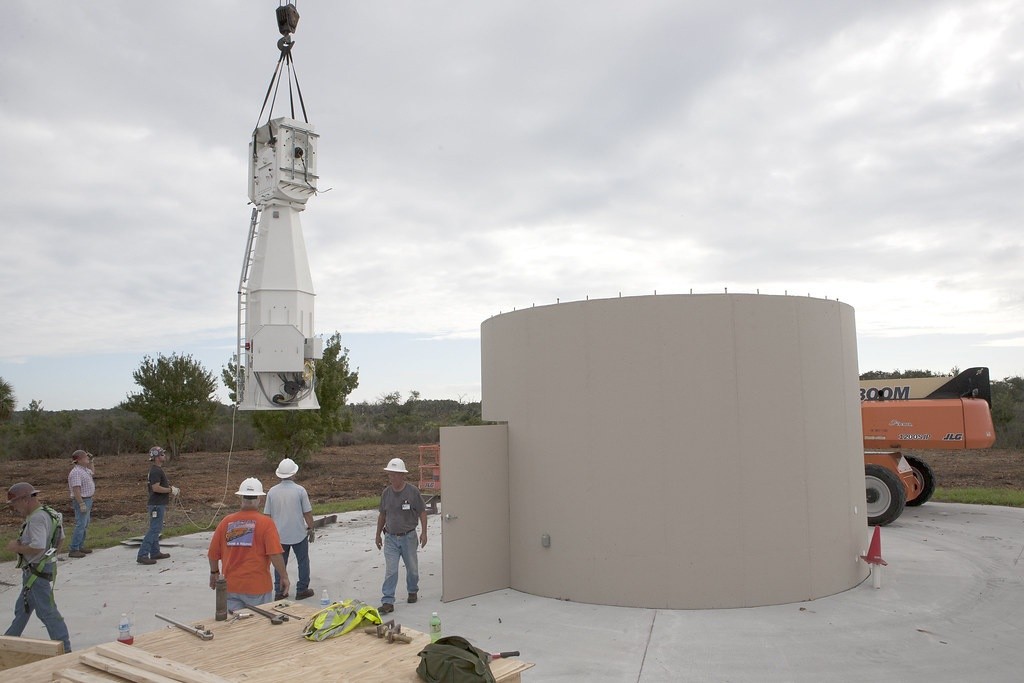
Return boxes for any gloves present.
[80,502,88,513]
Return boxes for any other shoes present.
[68,550,87,558]
[136,557,157,565]
[378,602,395,613]
[79,547,93,554]
[406,592,419,603]
[274,592,290,602]
[295,588,316,600]
[153,552,171,559]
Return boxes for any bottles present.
[118,613,130,640]
[429,611,441,643]
[320,590,330,609]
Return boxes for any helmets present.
[7,482,41,504]
[233,478,267,496]
[147,446,166,462]
[383,457,410,472]
[70,450,88,465]
[275,458,298,479]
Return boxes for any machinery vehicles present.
[857,367,998,527]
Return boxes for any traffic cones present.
[859,525,889,567]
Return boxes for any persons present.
[208,477,290,616]
[262,458,316,600]
[375,458,427,612]
[4,481,74,654]
[137,445,181,565]
[68,450,97,558]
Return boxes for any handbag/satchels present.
[416,635,497,683]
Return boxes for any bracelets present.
[210,570,219,574]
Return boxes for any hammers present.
[486,651,520,663]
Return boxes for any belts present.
[385,528,417,536]
[82,497,92,500]
